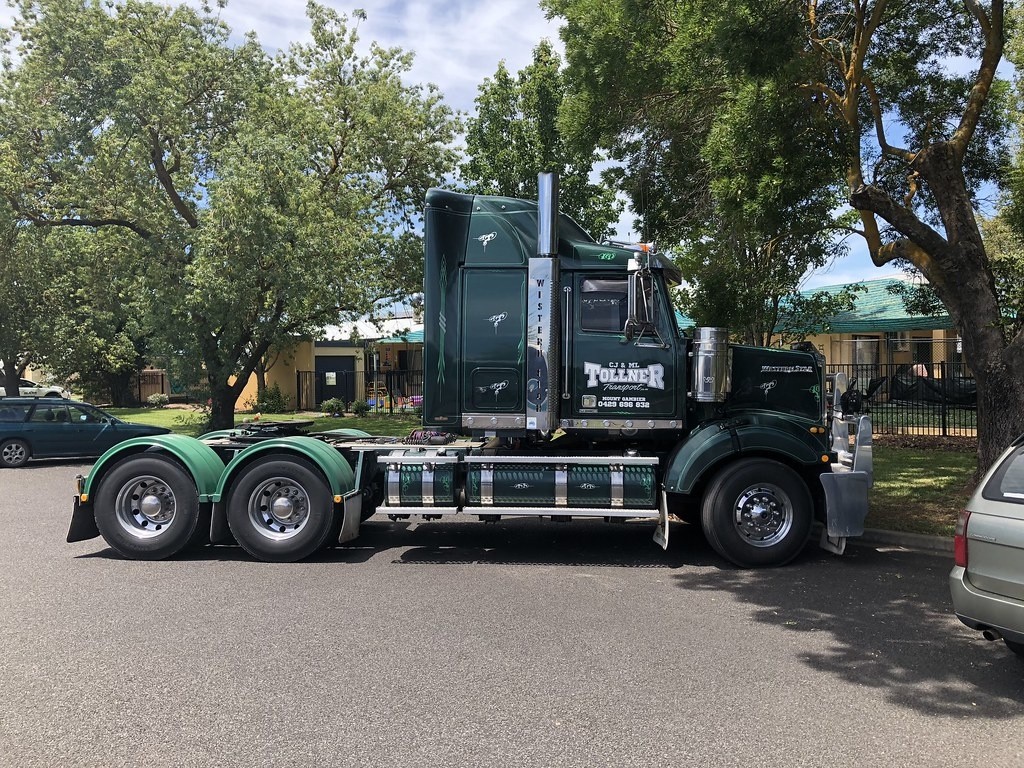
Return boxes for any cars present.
[950,433,1024,657]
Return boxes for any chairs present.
[0,407,69,424]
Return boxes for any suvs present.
[0,378,174,468]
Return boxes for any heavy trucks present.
[66,172,874,569]
